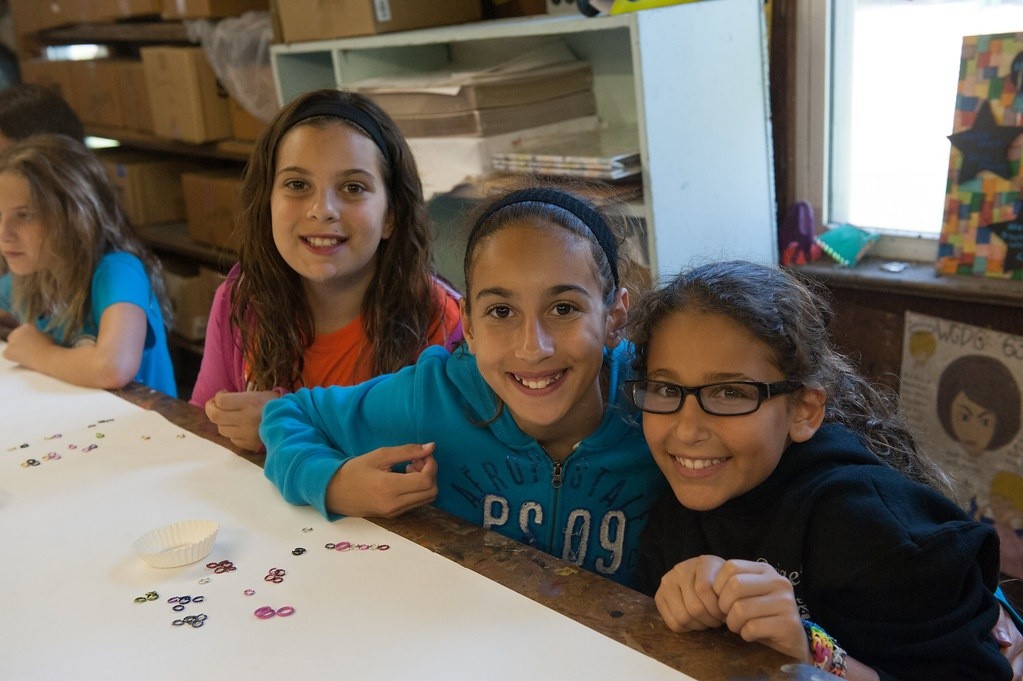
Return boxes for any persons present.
[2,135,181,402]
[4,77,89,175]
[253,181,1023,678]
[183,86,471,458]
[619,254,1018,681]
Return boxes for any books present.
[473,127,640,218]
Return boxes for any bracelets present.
[803,615,849,679]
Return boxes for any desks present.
[1,339,845,681]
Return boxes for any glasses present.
[627,377,803,417]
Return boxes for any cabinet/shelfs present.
[266,0,783,310]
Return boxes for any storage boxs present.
[0,1,483,358]
